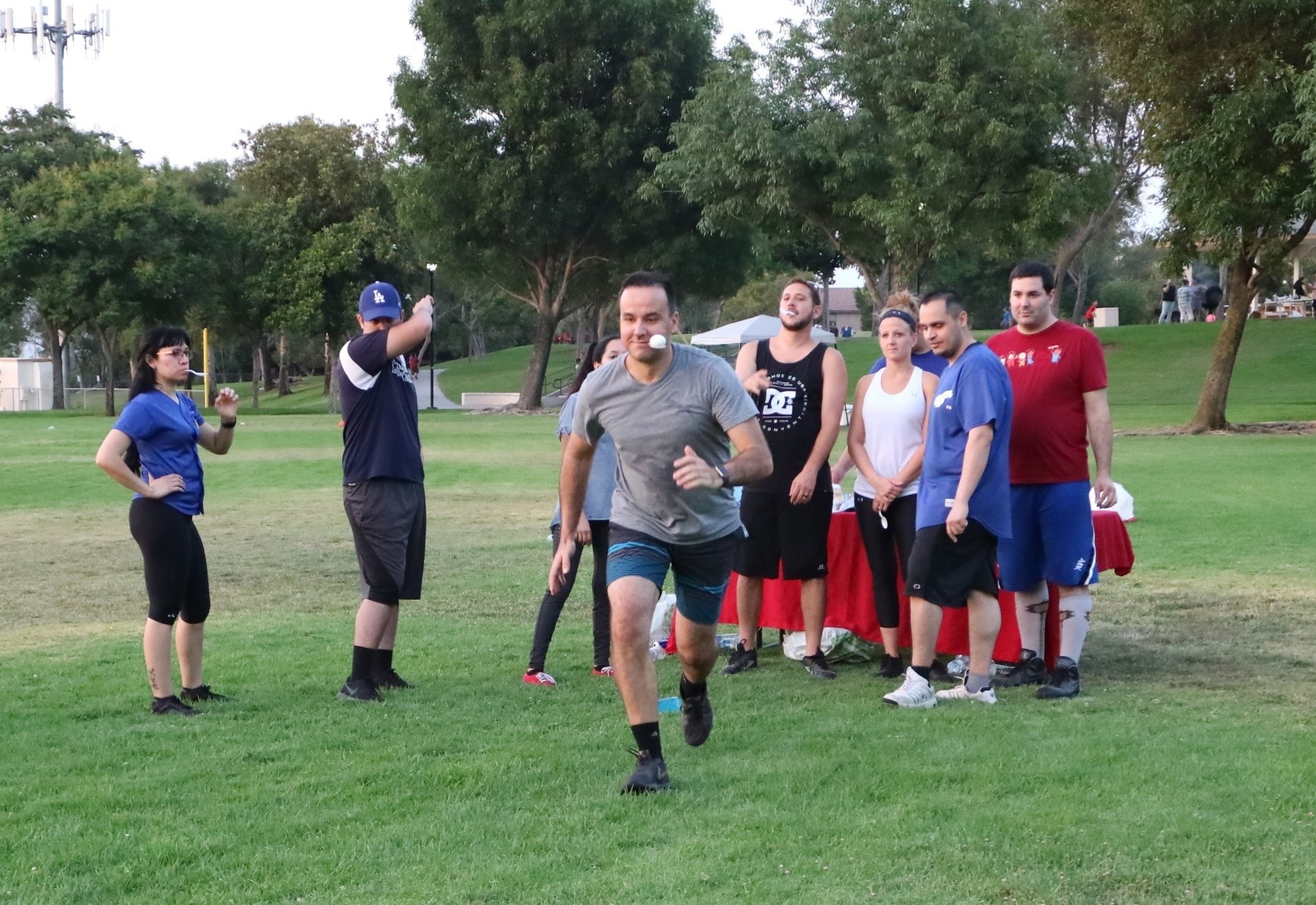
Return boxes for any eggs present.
[649,334,666,347]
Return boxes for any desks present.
[720,510,1140,678]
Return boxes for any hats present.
[359,281,402,322]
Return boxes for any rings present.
[803,494,807,497]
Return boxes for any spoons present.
[647,343,666,349]
[879,510,888,529]
[185,369,209,377]
[780,307,796,315]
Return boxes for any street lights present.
[426,263,438,408]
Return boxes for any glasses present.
[154,348,192,361]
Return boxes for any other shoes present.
[875,652,903,678]
[928,659,955,683]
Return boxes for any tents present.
[691,314,837,354]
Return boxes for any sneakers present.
[593,667,614,677]
[620,748,670,794]
[180,684,231,703]
[725,639,758,674]
[803,646,837,679]
[1035,656,1080,698]
[680,690,713,746]
[151,697,202,715]
[935,675,997,703]
[993,648,1046,688]
[523,672,557,686]
[374,670,414,688]
[336,676,384,703]
[882,666,937,708]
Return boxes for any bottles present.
[658,697,681,712]
[650,646,666,662]
[947,655,971,674]
[718,637,737,650]
[715,634,738,642]
[988,664,1014,675]
[652,640,667,648]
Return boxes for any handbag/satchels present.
[783,626,878,664]
[649,591,678,646]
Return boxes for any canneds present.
[990,664,1010,675]
[652,640,666,649]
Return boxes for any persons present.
[96,325,239,717]
[717,277,837,682]
[1084,299,1098,327]
[879,290,1013,710]
[845,291,951,680]
[1158,272,1316,324]
[815,319,839,337]
[554,330,575,344]
[831,310,950,487]
[335,279,435,704]
[982,260,1117,699]
[547,269,773,796]
[1000,307,1013,330]
[409,352,419,380]
[521,337,616,686]
[575,358,581,369]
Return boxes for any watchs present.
[220,415,237,429]
[716,464,730,488]
